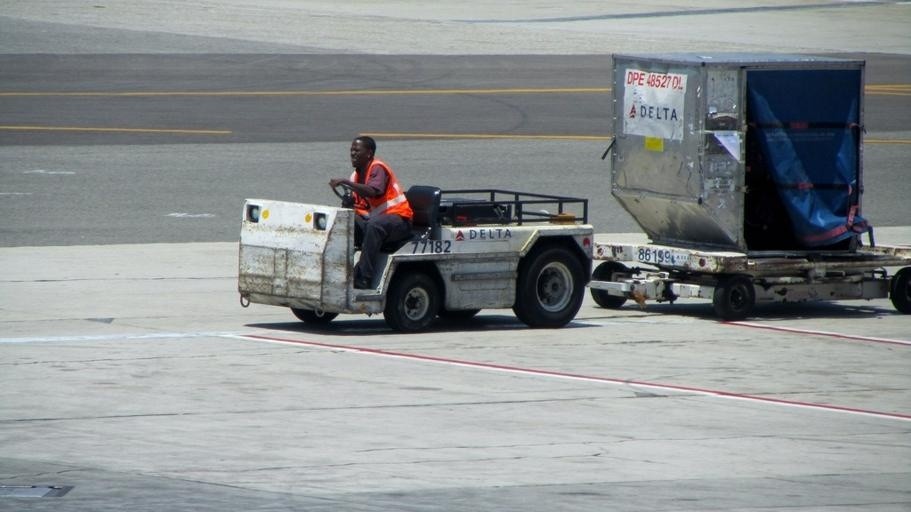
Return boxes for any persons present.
[331,136,415,287]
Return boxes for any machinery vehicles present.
[238,178,594,334]
